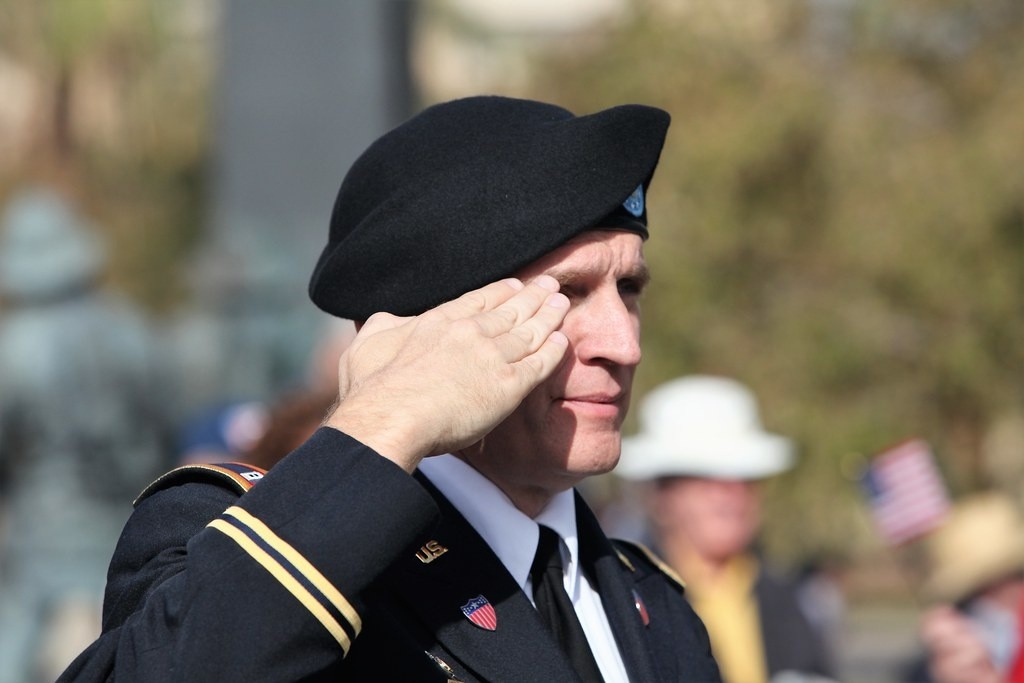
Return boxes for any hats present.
[921,489,1024,607]
[607,371,804,483]
[308,94,672,324]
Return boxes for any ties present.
[522,523,608,683]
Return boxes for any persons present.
[54,97,726,683]
[900,489,1024,683]
[608,373,839,683]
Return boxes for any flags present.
[854,434,946,549]
